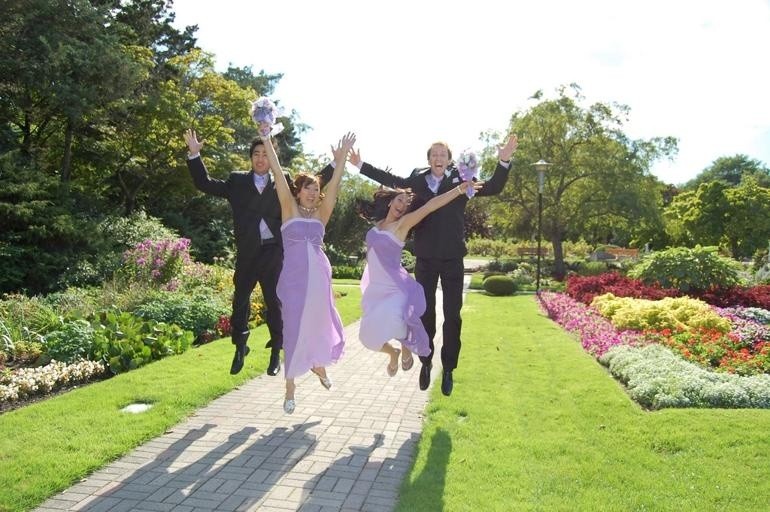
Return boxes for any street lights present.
[530,159,553,295]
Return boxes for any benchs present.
[518,247,548,259]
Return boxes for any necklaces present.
[298,204,317,219]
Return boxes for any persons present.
[255,123,357,415]
[356,164,485,379]
[181,127,342,378]
[344,133,520,395]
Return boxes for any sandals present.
[401,343,413,370]
[284,386,296,415]
[387,348,400,377]
[311,369,331,389]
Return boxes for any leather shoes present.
[441,369,453,397]
[419,362,433,390]
[267,357,281,376]
[230,346,250,375]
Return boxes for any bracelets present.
[260,133,271,142]
[501,159,510,164]
[456,185,464,195]
